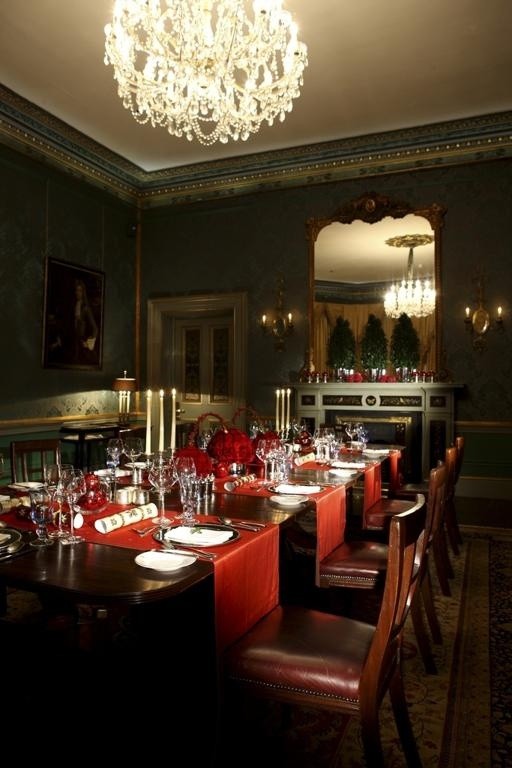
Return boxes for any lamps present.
[462,269,505,356]
[378,233,440,320]
[101,0,316,150]
[259,274,297,353]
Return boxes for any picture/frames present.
[40,251,108,375]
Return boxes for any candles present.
[142,386,156,459]
[118,389,131,415]
[280,389,286,429]
[286,388,291,429]
[157,389,166,454]
[169,387,178,450]
[274,389,280,431]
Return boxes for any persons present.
[49,276,98,365]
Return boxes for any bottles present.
[306,371,329,383]
[413,370,435,384]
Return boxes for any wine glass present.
[28,421,369,547]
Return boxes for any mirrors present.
[304,191,448,379]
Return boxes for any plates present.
[135,548,198,575]
[1,480,45,562]
[132,564,196,582]
